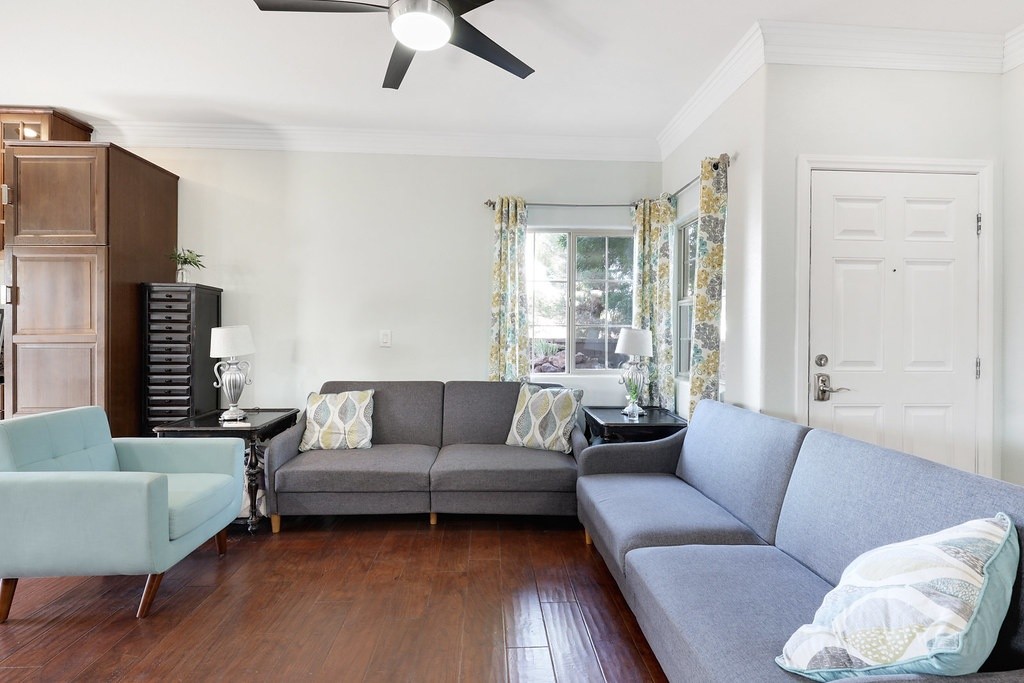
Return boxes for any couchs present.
[576,398,1024,683]
[264,380,590,533]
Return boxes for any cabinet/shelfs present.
[0,106,219,437]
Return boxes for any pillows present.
[775,512,1019,681]
[299,388,375,451]
[505,380,584,455]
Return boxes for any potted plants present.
[626,376,641,421]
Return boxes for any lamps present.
[210,324,255,422]
[614,328,652,414]
[388,0,455,53]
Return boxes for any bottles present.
[628,403,638,420]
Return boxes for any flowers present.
[165,248,205,280]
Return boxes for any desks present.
[582,405,688,447]
[153,407,299,536]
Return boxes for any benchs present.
[0,407,246,624]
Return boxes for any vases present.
[176,264,190,283]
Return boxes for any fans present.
[253,0,535,90]
